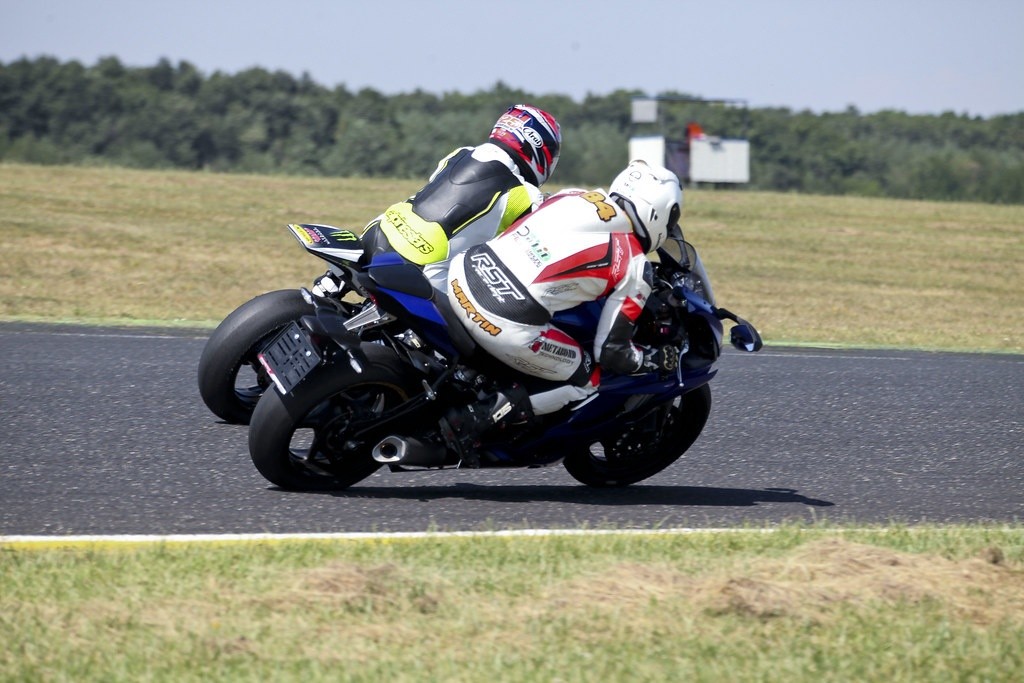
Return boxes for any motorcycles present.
[195,219,761,492]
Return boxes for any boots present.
[444,390,532,470]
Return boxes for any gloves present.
[639,344,681,376]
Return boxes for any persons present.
[447,159,684,439]
[311,102,561,301]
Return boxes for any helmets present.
[489,103,562,188]
[608,159,683,254]
